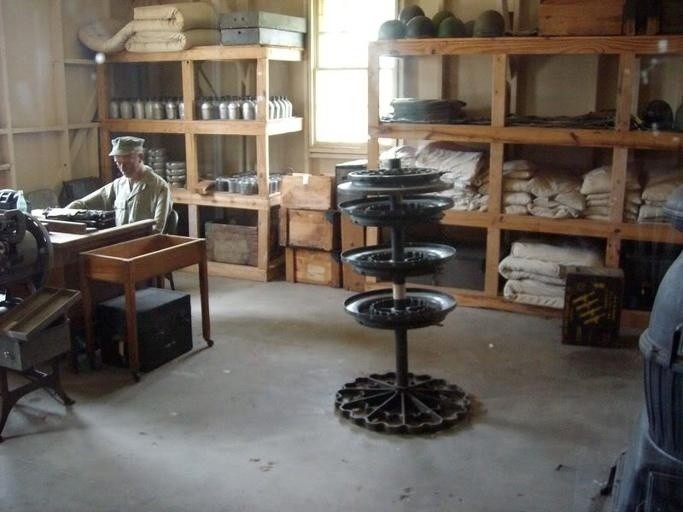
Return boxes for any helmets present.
[675,105,683,131]
[437,17,466,38]
[378,20,405,40]
[645,100,673,128]
[465,20,474,37]
[432,10,455,33]
[400,5,425,27]
[474,10,505,38]
[405,16,437,38]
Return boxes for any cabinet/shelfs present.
[95,45,308,281]
[367,36,683,328]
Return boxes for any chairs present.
[163,210,179,290]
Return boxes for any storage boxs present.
[561,265,625,348]
[205,160,365,293]
[0,286,81,371]
[97,286,194,372]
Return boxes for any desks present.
[74,231,214,383]
[23,208,162,375]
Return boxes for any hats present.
[108,135,145,156]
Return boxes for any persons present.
[66,136,170,235]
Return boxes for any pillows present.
[478,160,683,229]
